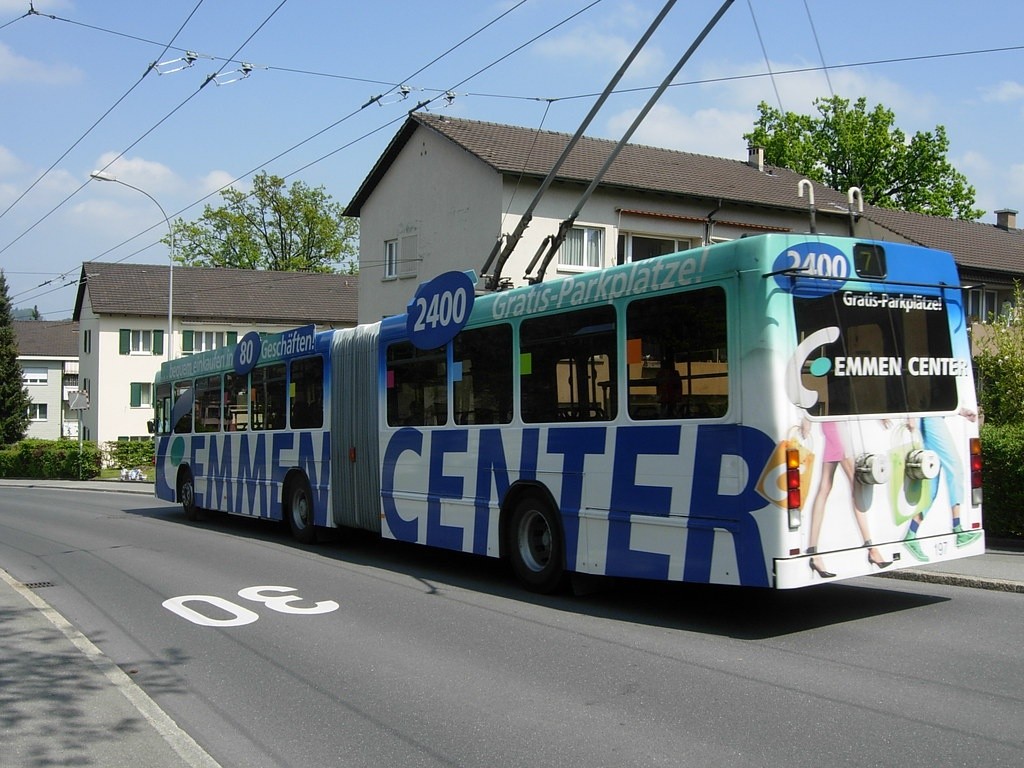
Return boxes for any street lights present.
[89,166,176,366]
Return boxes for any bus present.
[146,232,988,618]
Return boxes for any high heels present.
[809,558,837,578]
[868,553,894,569]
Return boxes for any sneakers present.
[904,530,930,562]
[953,525,982,548]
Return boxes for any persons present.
[174,383,323,434]
[799,407,981,579]
[657,361,682,396]
